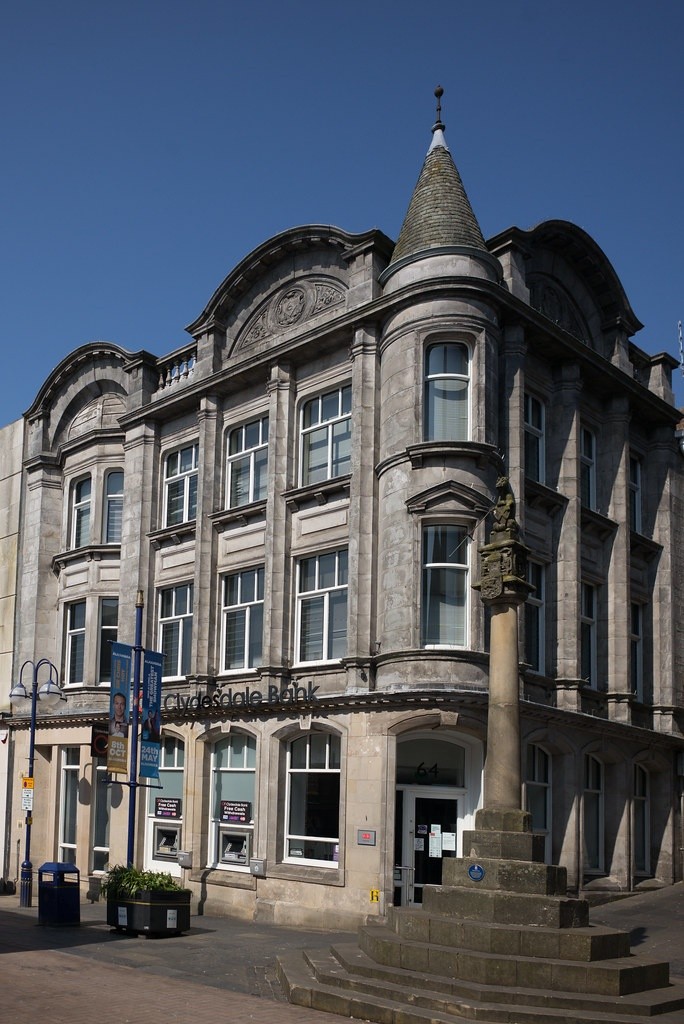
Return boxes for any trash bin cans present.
[38,862,80,927]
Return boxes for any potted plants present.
[98,861,193,939]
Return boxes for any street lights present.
[8,658,68,908]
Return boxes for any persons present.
[143,709,159,738]
[112,722,124,738]
[109,693,128,738]
